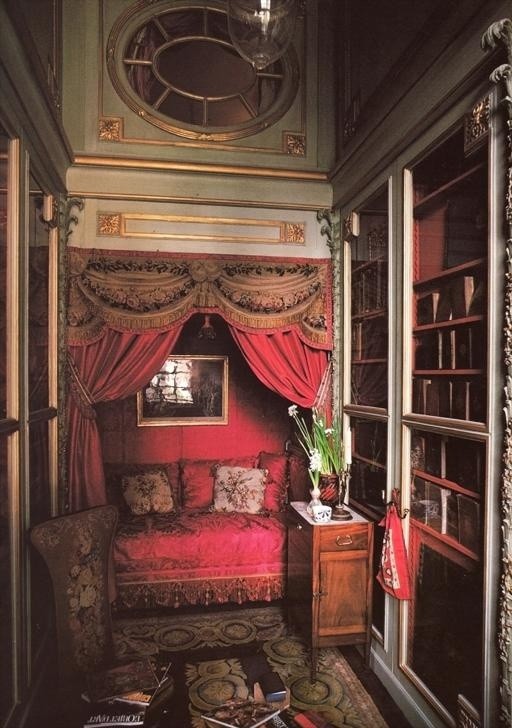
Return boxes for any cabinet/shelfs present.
[340,70,502,727]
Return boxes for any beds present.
[108,444,314,614]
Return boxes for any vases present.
[318,473,345,510]
[306,487,323,520]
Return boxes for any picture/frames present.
[135,352,231,427]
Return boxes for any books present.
[349,217,387,505]
[411,277,489,560]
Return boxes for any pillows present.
[102,450,289,525]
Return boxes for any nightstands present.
[284,499,374,683]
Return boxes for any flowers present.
[309,405,343,474]
[287,403,320,487]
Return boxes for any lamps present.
[226,0,299,71]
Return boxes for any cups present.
[312,506,332,523]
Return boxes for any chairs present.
[25,503,183,726]
